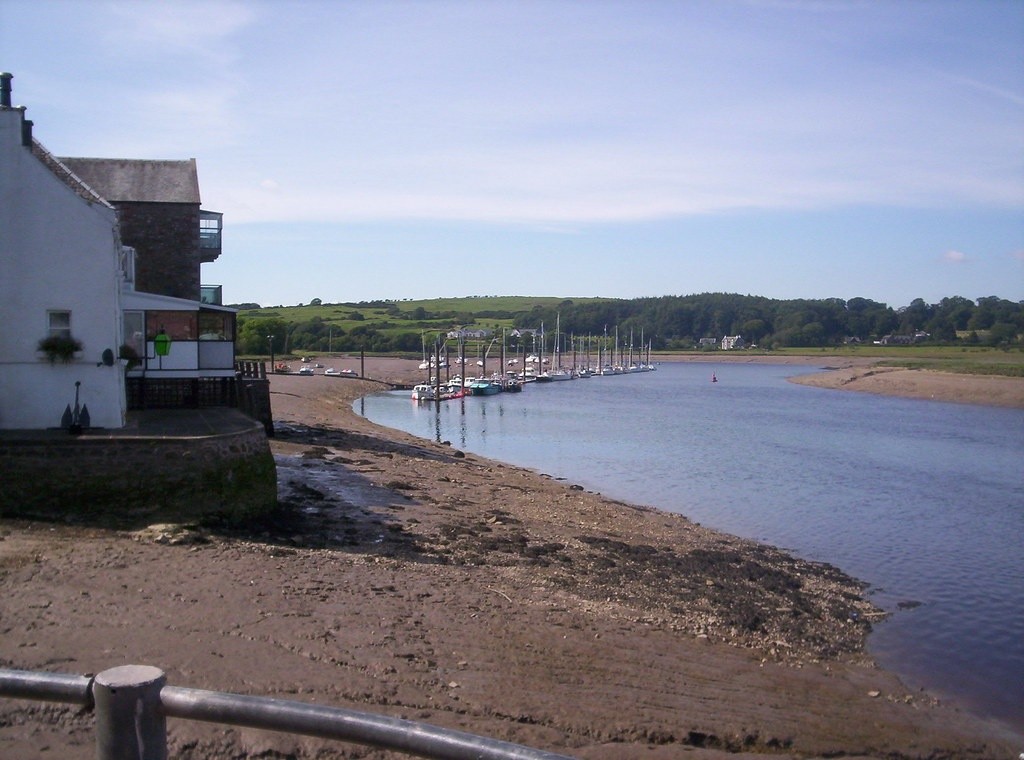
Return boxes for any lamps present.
[117,324,171,359]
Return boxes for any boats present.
[277,357,359,377]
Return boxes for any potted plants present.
[36,335,85,368]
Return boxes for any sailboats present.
[411,308,657,400]
